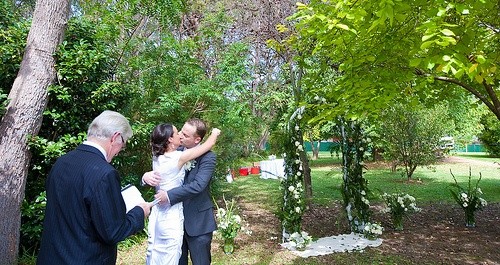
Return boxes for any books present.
[120,184,162,214]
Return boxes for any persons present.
[141,118,218,264]
[36,110,154,265]
[146,124,220,265]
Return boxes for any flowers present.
[364,222,383,239]
[291,230,312,250]
[446,168,488,224]
[212,194,242,252]
[380,189,415,229]
[184,161,196,172]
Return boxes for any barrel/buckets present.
[239,168,248,175]
[251,167,260,174]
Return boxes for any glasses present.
[120,134,127,151]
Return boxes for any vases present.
[368,232,377,242]
[224,238,234,255]
[391,214,405,232]
[296,242,307,251]
[464,210,478,231]
